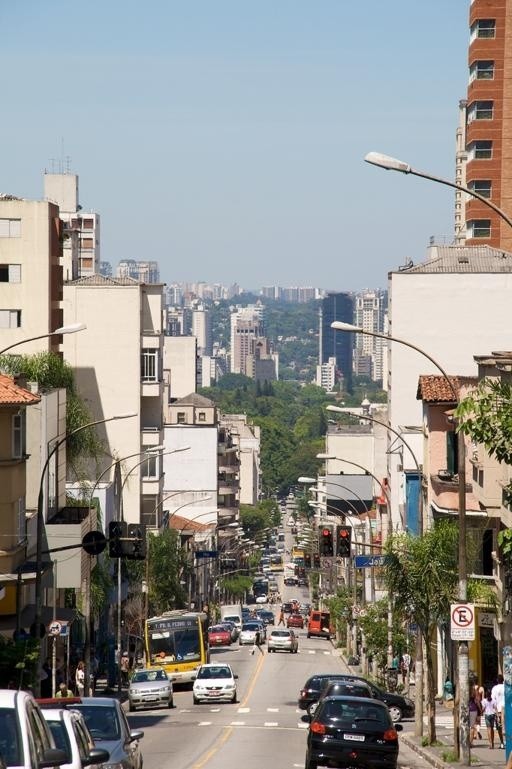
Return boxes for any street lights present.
[282,472,376,656]
[115,444,192,668]
[82,444,164,692]
[317,451,397,666]
[330,319,473,765]
[0,321,87,355]
[35,411,140,627]
[326,401,424,731]
[364,150,512,236]
[146,484,280,611]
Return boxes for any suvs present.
[316,681,372,710]
[300,675,419,723]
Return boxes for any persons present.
[278,609,286,626]
[250,628,265,657]
[118,649,131,686]
[445,670,506,751]
[268,592,282,611]
[393,647,411,685]
[38,645,98,700]
[303,613,310,627]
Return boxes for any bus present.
[144,609,210,688]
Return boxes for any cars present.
[65,697,145,769]
[209,488,332,654]
[33,697,110,769]
[190,660,240,704]
[305,696,404,767]
[0,689,67,769]
[127,667,177,708]
